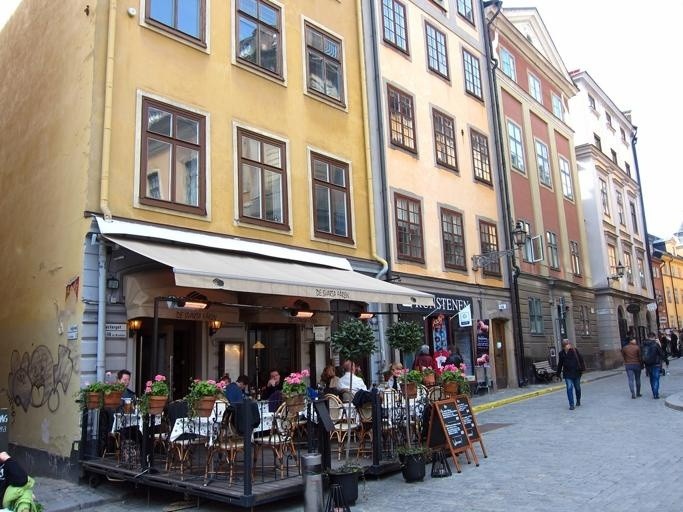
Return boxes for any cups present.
[123,398,131,413]
[250,390,255,400]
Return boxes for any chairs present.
[81,379,445,486]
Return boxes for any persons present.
[626,329,683,376]
[113,344,464,474]
[556,338,586,409]
[0,450,28,511]
[641,332,668,398]
[621,337,644,399]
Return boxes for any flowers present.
[136,375,168,413]
[278,369,310,398]
[420,365,435,376]
[391,368,419,384]
[437,364,473,396]
[101,371,128,395]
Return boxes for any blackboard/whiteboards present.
[434,394,481,454]
[0,407,10,481]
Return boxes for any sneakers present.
[637,391,642,397]
[632,394,636,399]
[569,404,574,410]
[576,402,580,406]
[654,394,660,399]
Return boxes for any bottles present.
[256,388,261,400]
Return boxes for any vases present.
[441,381,460,397]
[421,373,436,388]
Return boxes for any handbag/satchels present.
[577,362,584,378]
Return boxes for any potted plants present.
[326,460,368,506]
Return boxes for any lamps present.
[471,219,531,273]
[607,262,624,286]
[354,312,376,321]
[126,316,142,336]
[177,298,208,312]
[288,309,315,321]
[207,314,221,336]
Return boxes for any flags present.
[458,303,472,327]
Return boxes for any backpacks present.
[641,345,657,365]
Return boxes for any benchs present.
[531,360,562,382]
